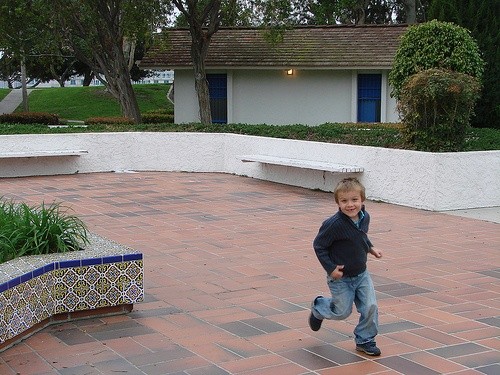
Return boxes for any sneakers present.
[356,341,382,356]
[308,301,323,332]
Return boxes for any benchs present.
[0,149,89,159]
[241,155,364,176]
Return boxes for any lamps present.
[287,69,293,75]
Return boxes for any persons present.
[308,177,384,356]
